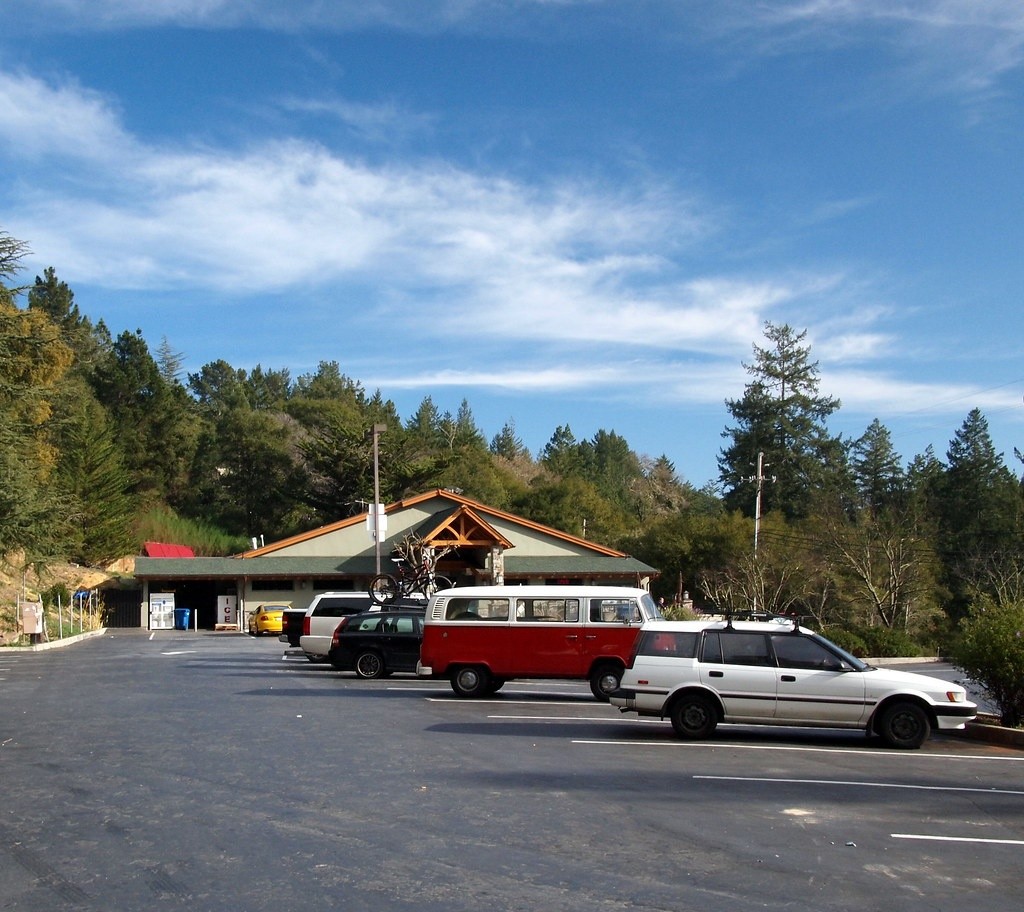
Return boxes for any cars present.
[248,602,292,637]
[327,608,427,680]
[609,609,978,749]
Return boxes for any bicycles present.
[369,541,454,605]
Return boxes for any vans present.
[416,583,678,703]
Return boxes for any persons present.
[657,597,665,611]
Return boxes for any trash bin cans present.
[174,607,190,630]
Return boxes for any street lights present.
[368,423,388,574]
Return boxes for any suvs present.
[300,590,470,664]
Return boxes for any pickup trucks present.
[279,608,307,647]
